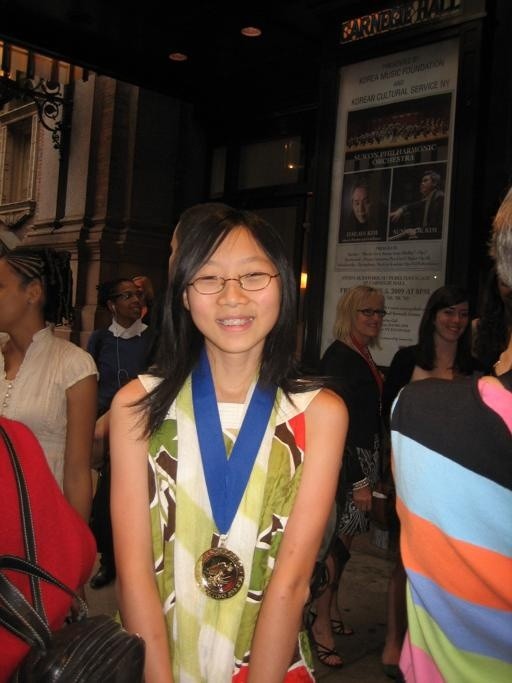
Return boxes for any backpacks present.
[0,426,143,683]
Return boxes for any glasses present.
[188,272,279,294]
[357,308,387,317]
[116,292,146,300]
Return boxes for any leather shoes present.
[383,663,406,682]
[90,566,113,589]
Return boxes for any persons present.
[0,243,102,621]
[306,283,386,668]
[389,189,512,683]
[108,206,351,683]
[471,266,511,361]
[84,275,157,590]
[391,171,444,239]
[132,273,154,316]
[91,201,236,441]
[350,179,386,241]
[0,414,101,680]
[378,285,474,682]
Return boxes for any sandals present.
[308,608,353,667]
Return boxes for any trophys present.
[193,545,245,600]
